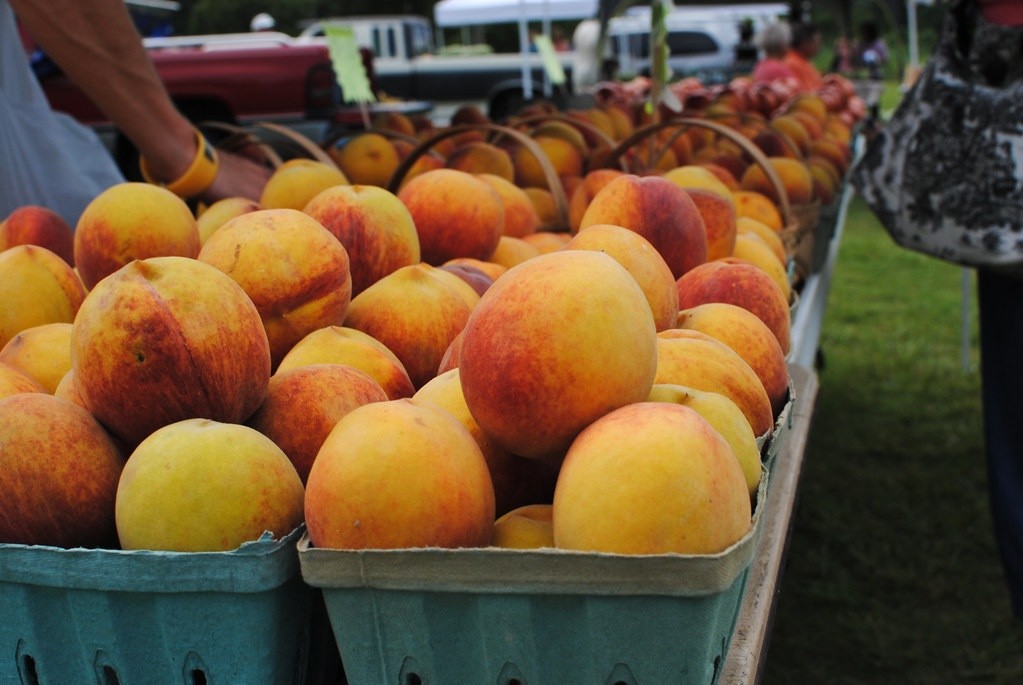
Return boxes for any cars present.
[30,0,800,186]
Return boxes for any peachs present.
[0,97,852,556]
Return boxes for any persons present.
[967,0,1023,623]
[734,20,891,78]
[0,0,272,229]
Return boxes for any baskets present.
[0,123,867,685]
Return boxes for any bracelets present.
[141,127,220,198]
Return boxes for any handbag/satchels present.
[852,43,1023,264]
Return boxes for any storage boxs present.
[0,120,869,684]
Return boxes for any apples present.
[452,74,868,127]
